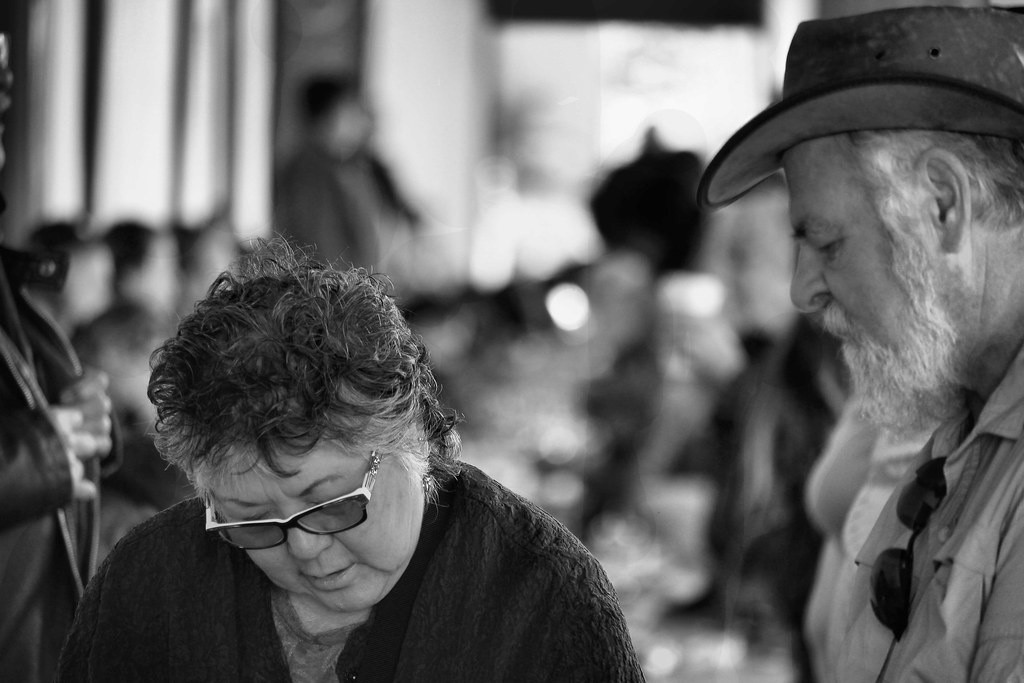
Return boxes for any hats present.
[697,6,1024,215]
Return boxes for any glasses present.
[871,456,946,641]
[204,449,385,550]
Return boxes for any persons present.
[700,8,1023,683]
[0,32,122,683]
[279,73,383,277]
[26,219,197,572]
[53,259,643,683]
